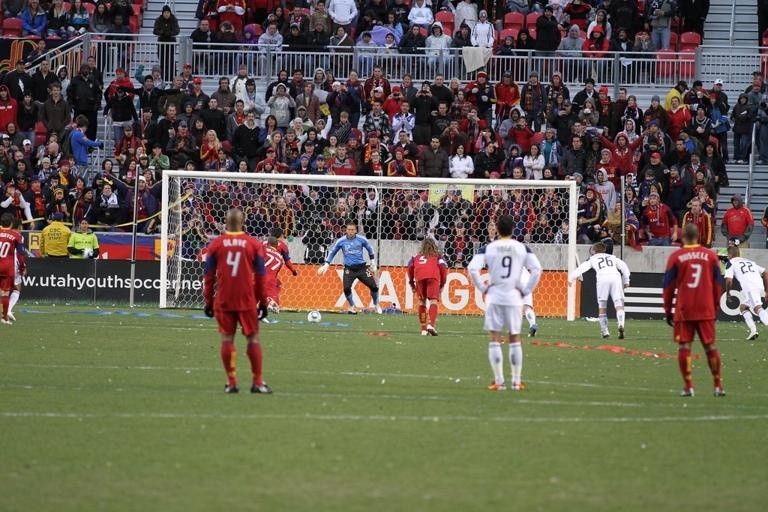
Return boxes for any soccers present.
[308,311,321,323]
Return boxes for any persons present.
[722,243,768,341]
[0,213,28,324]
[1,0,768,257]
[660,222,727,397]
[6,219,24,322]
[404,235,448,336]
[465,213,544,392]
[314,221,383,316]
[566,240,631,341]
[259,235,285,324]
[200,207,275,395]
[516,266,538,339]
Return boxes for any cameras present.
[373,90,378,93]
[482,132,487,136]
[394,94,399,96]
[637,36,642,40]
[14,193,20,204]
[516,125,521,128]
[627,109,633,118]
[223,24,232,30]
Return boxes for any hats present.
[14,59,25,65]
[477,69,608,95]
[680,127,690,135]
[1,134,94,200]
[678,79,724,88]
[139,175,146,182]
[267,141,325,161]
[194,78,201,84]
[394,86,400,92]
[179,121,187,128]
[650,95,661,158]
[184,62,192,69]
[152,65,161,71]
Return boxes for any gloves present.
[258,306,267,321]
[317,262,329,276]
[370,260,377,273]
[204,306,214,318]
[293,270,298,276]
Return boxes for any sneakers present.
[745,331,759,340]
[680,387,693,396]
[251,383,274,394]
[617,323,625,339]
[422,330,431,336]
[225,384,239,392]
[427,324,438,336]
[268,304,279,316]
[349,308,357,314]
[375,305,383,314]
[487,382,507,391]
[512,381,525,390]
[600,330,610,338]
[7,312,16,322]
[1,318,14,325]
[267,297,279,310]
[527,324,537,337]
[714,386,726,397]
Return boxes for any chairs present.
[420,9,608,49]
[1,0,141,48]
[654,31,703,77]
